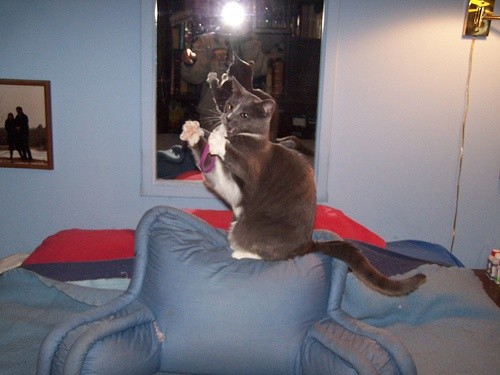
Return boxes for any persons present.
[181,22,268,132]
[5,106,32,160]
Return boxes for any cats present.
[180,72,428,296]
[206,52,274,115]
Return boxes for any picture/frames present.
[0,78,54,170]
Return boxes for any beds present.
[0,204,500,375]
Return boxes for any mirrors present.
[141,0,338,200]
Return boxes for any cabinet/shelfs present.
[169,0,292,102]
